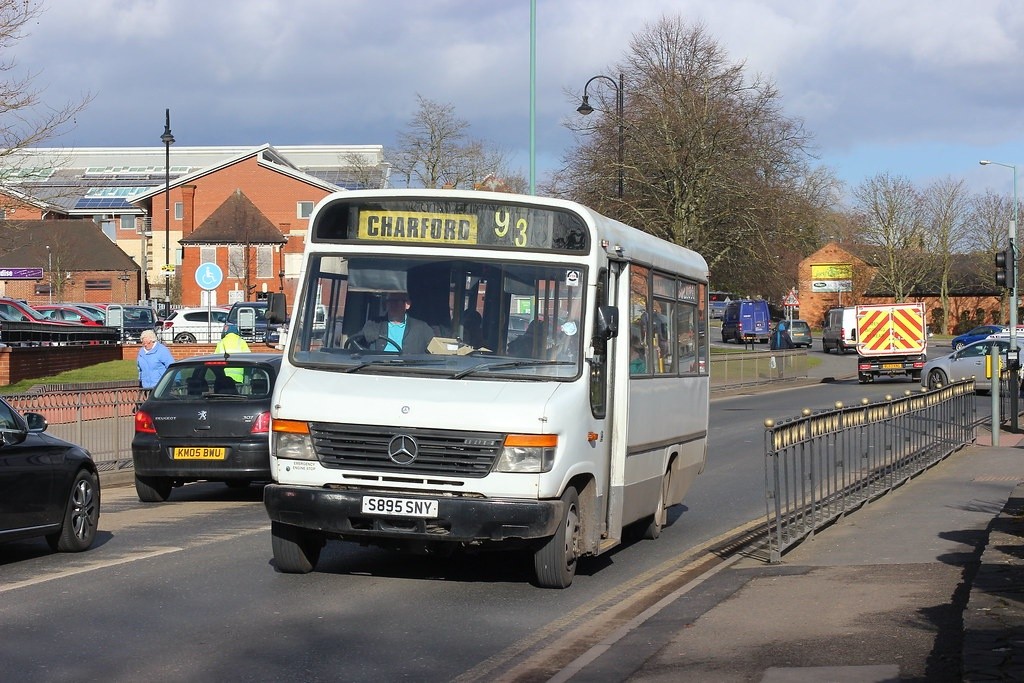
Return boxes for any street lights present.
[160,106,178,318]
[577,74,632,214]
[979,159,1020,434]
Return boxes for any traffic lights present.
[994,247,1017,292]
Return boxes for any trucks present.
[855,302,930,383]
[719,300,772,343]
[821,306,857,355]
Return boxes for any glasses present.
[384,296,407,303]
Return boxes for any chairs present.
[189,367,268,395]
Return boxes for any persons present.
[214,326,251,383]
[508,319,559,360]
[771,321,794,378]
[462,309,481,344]
[630,336,645,373]
[137,330,181,397]
[363,292,433,354]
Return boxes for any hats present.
[226,326,238,334]
[630,324,642,348]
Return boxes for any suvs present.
[219,301,283,345]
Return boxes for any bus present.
[707,291,741,319]
[262,185,712,586]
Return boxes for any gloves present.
[176,382,181,387]
[139,382,143,388]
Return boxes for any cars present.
[0,295,175,345]
[159,308,230,345]
[131,329,285,505]
[920,338,1024,397]
[509,313,567,345]
[951,324,1007,351]
[0,397,100,562]
[769,319,813,349]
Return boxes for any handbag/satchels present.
[132,400,142,413]
